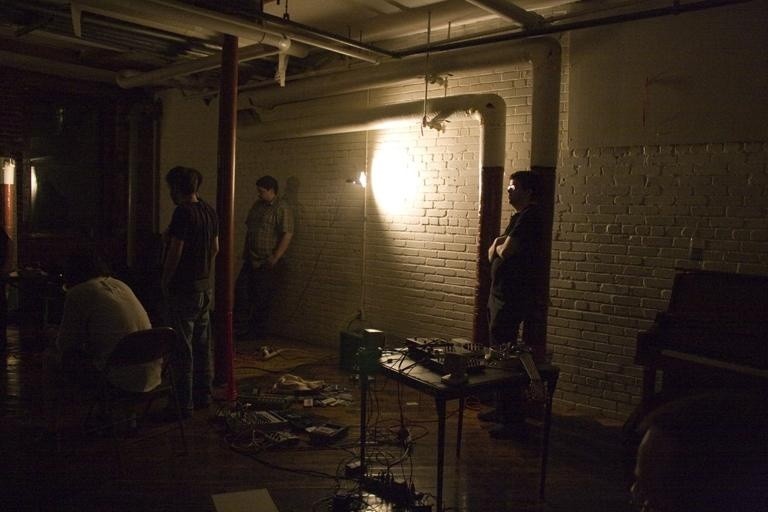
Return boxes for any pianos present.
[636,268,767,415]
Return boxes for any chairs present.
[78,325,194,473]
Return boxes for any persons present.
[32,253,165,445]
[622,390,768,511]
[476,170,545,424]
[234,173,293,341]
[147,165,216,423]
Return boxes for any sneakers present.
[478,407,529,439]
[164,393,212,419]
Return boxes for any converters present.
[345,460,361,476]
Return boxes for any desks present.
[356,339,562,510]
